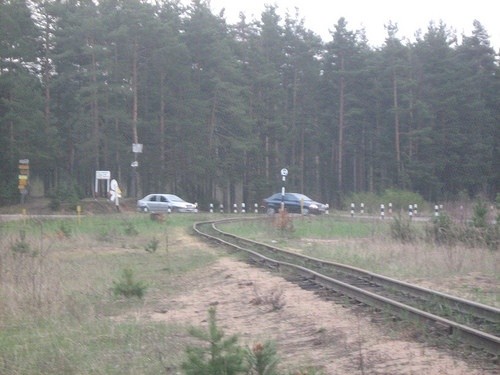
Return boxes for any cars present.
[262,192,327,216]
[136,193,198,214]
